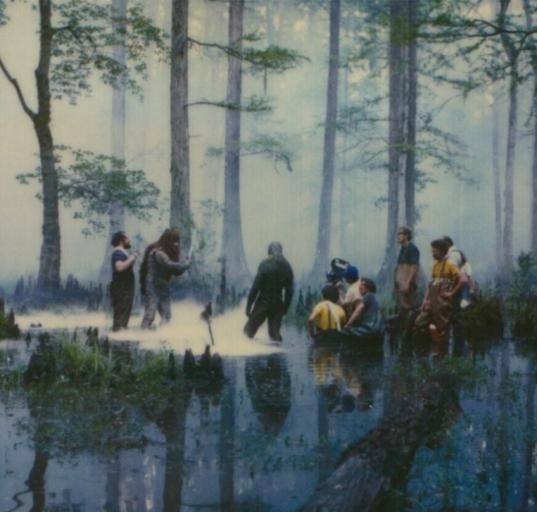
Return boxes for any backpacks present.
[326,256,358,282]
[455,274,481,319]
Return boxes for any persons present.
[111,230,136,331]
[310,348,374,411]
[140,229,192,327]
[244,240,293,341]
[308,226,473,356]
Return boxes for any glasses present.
[398,232,406,234]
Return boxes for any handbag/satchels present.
[323,326,345,355]
